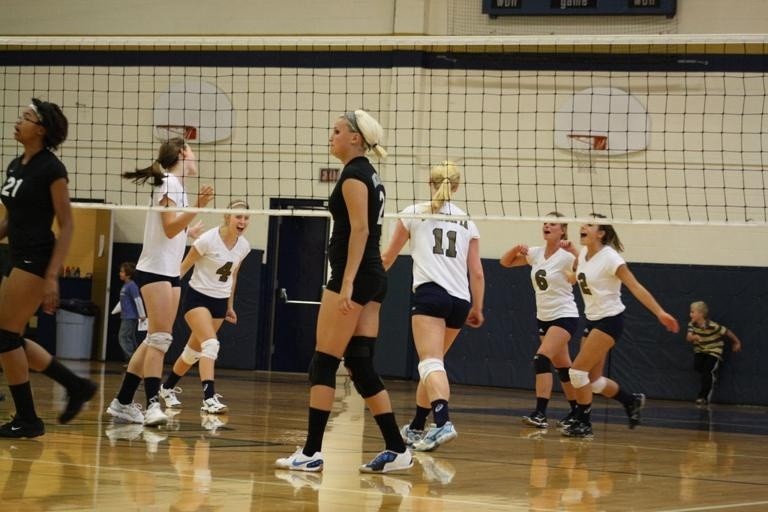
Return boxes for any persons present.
[273,110,414,472]
[687,302,741,405]
[158,200,251,415]
[117,262,146,367]
[380,160,485,451]
[0,97,99,438]
[499,212,578,428]
[106,137,214,426]
[556,213,679,437]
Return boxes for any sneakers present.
[106,398,145,423]
[1,418,45,437]
[626,394,645,430]
[401,425,423,446]
[556,415,579,428]
[411,422,457,451]
[158,386,182,408]
[57,379,98,423]
[275,448,324,472]
[561,422,593,438]
[143,402,168,426]
[201,396,228,415]
[522,412,549,429]
[359,448,415,473]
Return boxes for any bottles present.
[59,265,81,278]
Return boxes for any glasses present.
[16,112,43,125]
